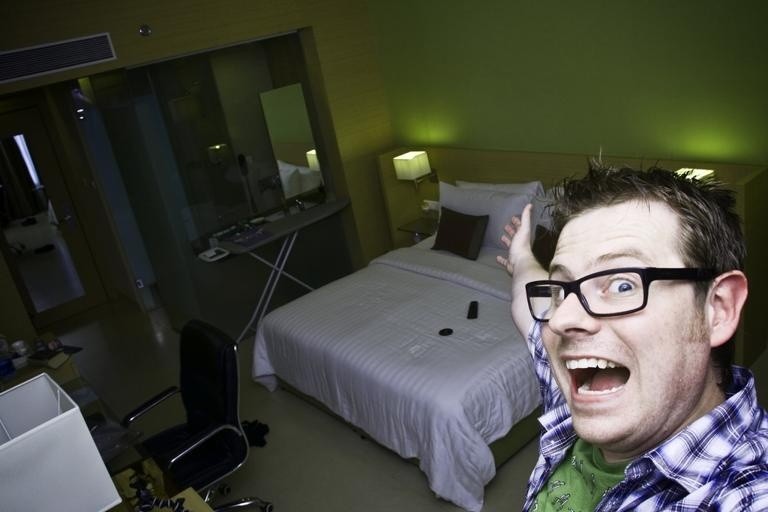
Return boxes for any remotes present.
[466,301,478,318]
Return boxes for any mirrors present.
[259,81,326,201]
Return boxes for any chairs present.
[120,320,274,512]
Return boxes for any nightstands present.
[398,217,436,245]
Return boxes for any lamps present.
[392,149,440,191]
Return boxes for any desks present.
[0,358,214,511]
[218,197,352,343]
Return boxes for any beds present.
[248,234,547,511]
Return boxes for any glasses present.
[525,268,718,322]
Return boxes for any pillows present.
[430,178,546,262]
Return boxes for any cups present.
[12,340,25,356]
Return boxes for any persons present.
[496,143,768,512]
